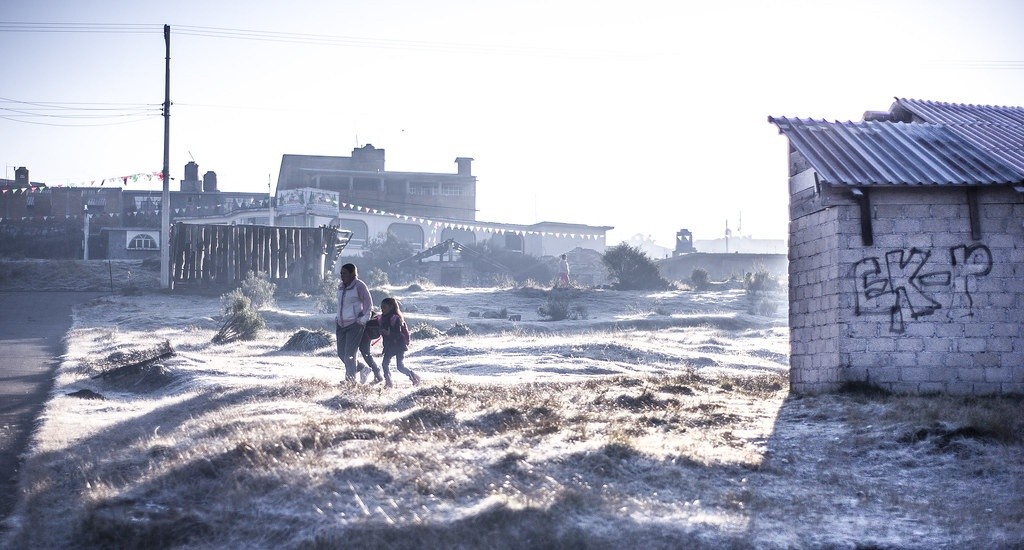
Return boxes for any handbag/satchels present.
[359,310,382,339]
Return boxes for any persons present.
[559,254,573,288]
[357,297,420,388]
[334,263,373,384]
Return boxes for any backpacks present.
[377,314,410,351]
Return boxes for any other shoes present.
[340,380,357,387]
[369,376,383,385]
[383,378,393,388]
[360,366,372,384]
[355,360,365,372]
[408,371,421,387]
